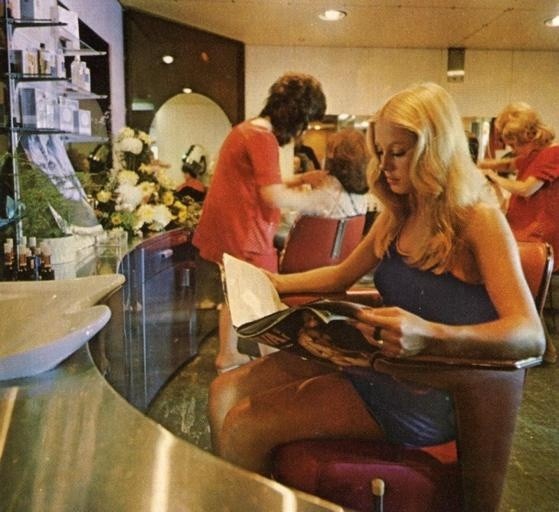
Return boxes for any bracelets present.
[300,172,307,184]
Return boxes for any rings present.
[373,326,386,347]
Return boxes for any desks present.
[303,124,374,170]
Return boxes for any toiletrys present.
[38,42,92,95]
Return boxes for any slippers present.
[217,355,256,376]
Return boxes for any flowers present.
[86,128,201,236]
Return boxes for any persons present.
[275,125,372,272]
[206,80,548,481]
[471,99,559,365]
[28,133,99,227]
[190,70,328,369]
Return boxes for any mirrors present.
[122,5,244,187]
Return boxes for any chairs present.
[293,145,320,175]
[475,117,518,161]
[278,213,379,307]
[267,240,554,511]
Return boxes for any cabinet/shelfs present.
[0,0,107,271]
